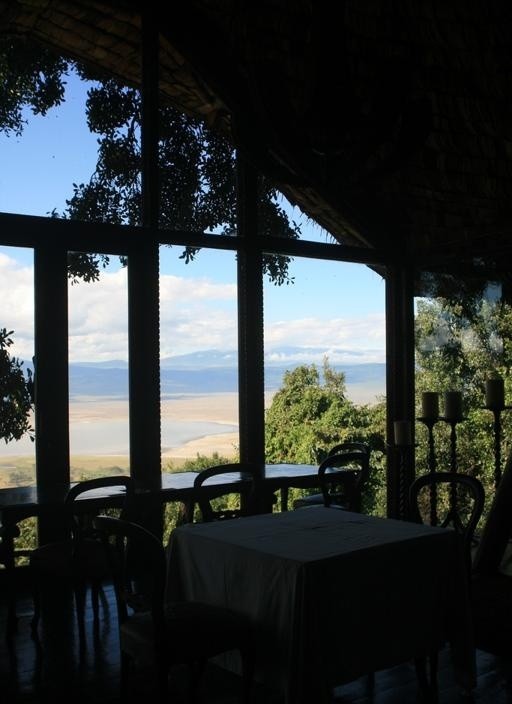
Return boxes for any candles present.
[444,391,461,419]
[422,391,438,417]
[485,379,504,406]
[393,420,411,445]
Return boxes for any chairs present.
[1,446,510,702]
